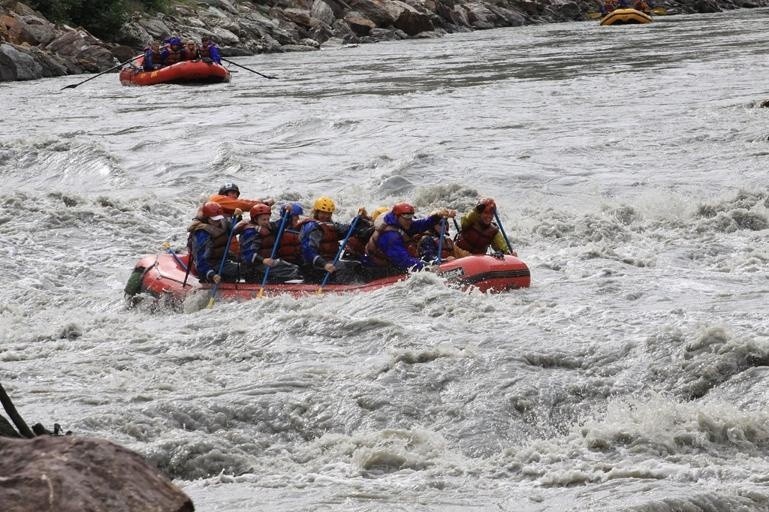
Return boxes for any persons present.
[296,198,368,285]
[417,220,472,264]
[187,183,276,256]
[274,203,303,264]
[405,209,456,257]
[341,206,389,260]
[239,203,303,283]
[452,197,517,257]
[141,32,220,73]
[187,201,242,286]
[363,202,449,282]
[601,0,651,16]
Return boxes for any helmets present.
[250,203,271,218]
[203,202,224,221]
[314,196,337,213]
[475,198,496,213]
[428,210,449,232]
[371,202,414,222]
[280,203,303,217]
[219,183,240,198]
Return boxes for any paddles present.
[220,57,279,80]
[650,8,669,14]
[60,46,169,90]
[590,12,609,19]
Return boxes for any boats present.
[600,9,653,26]
[127,252,531,313]
[118,54,229,85]
